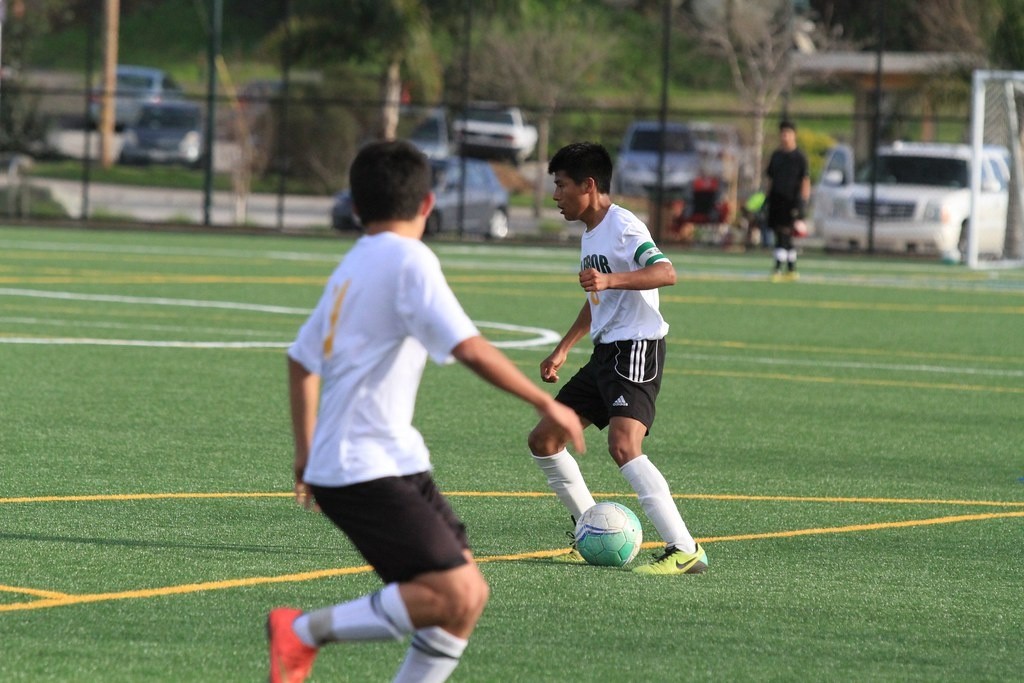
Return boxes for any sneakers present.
[268,608,319,683]
[553,515,587,563]
[632,542,709,575]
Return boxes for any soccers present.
[574,500,644,568]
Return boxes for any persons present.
[764,122,810,282]
[265,140,586,683]
[528,143,710,575]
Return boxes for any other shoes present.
[769,269,781,283]
[787,270,800,281]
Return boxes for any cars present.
[231,76,336,153]
[117,100,205,169]
[331,147,511,245]
[805,138,1013,268]
[607,120,758,217]
[87,62,188,135]
[450,100,550,165]
